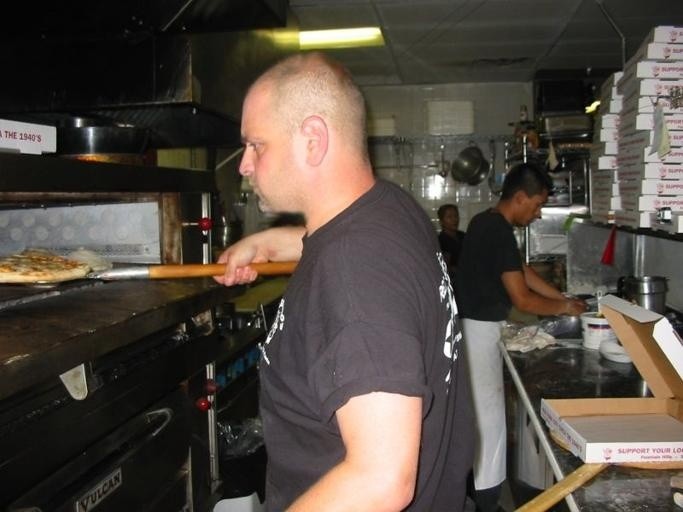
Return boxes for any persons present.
[210,49,477,512]
[459,162,591,512]
[437,204,465,262]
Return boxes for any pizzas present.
[0,252,91,284]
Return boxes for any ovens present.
[0,165,254,511]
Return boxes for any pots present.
[449,140,489,186]
[614,275,669,315]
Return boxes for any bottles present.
[519,104,528,120]
